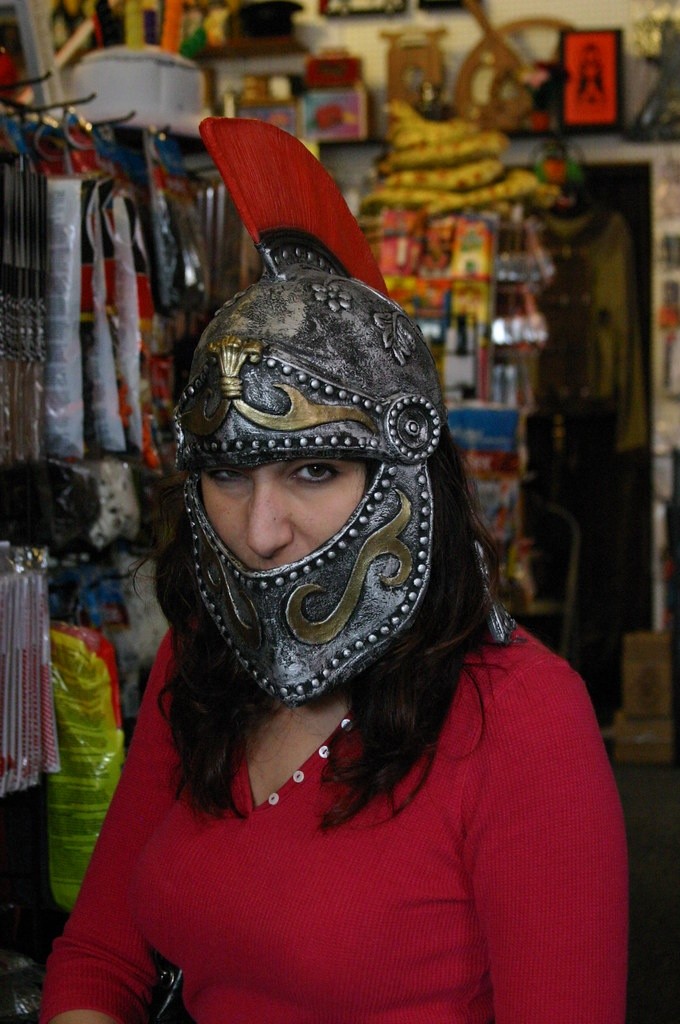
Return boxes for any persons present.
[39,114,631,1022]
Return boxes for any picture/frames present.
[558,26,627,136]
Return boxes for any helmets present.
[172,117,515,708]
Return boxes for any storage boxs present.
[614,710,675,768]
[624,632,673,720]
